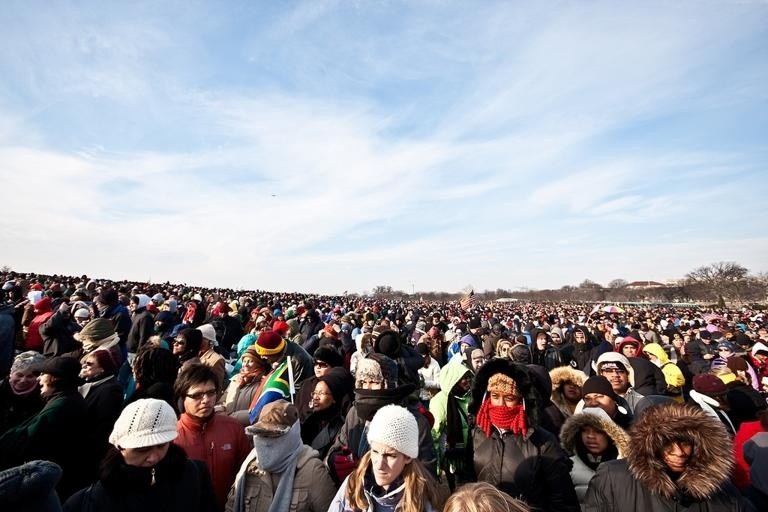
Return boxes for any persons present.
[4,271,768,512]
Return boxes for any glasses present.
[184,386,218,401]
[313,361,329,368]
[310,391,332,398]
[172,337,184,347]
[501,347,507,352]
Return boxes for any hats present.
[581,375,615,400]
[1,460,64,511]
[443,319,505,373]
[255,330,285,356]
[312,337,344,368]
[109,397,180,451]
[241,344,268,368]
[244,399,300,435]
[323,315,401,390]
[79,318,114,340]
[662,326,751,352]
[30,284,118,312]
[96,345,123,375]
[692,373,731,397]
[367,404,420,461]
[33,357,86,387]
